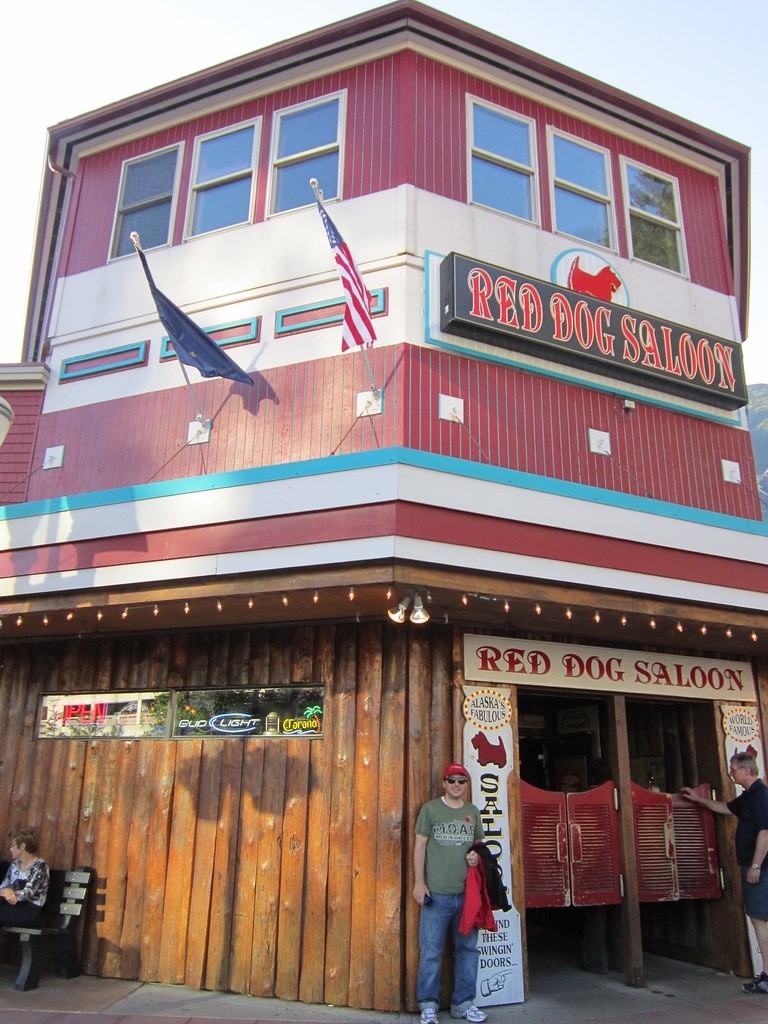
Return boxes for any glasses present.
[443,777,468,784]
[730,766,745,776]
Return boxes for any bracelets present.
[471,860,472,864]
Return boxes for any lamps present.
[387,590,431,623]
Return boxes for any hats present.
[444,763,469,779]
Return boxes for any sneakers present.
[421,1007,438,1024]
[742,972,768,994]
[451,1005,488,1022]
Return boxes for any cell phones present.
[424,894,433,906]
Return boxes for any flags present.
[318,202,376,353]
[136,247,255,386]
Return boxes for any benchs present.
[0,864,95,991]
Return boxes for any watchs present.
[751,864,761,869]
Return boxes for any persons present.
[413,765,486,1024]
[680,752,768,992]
[0,829,50,927]
